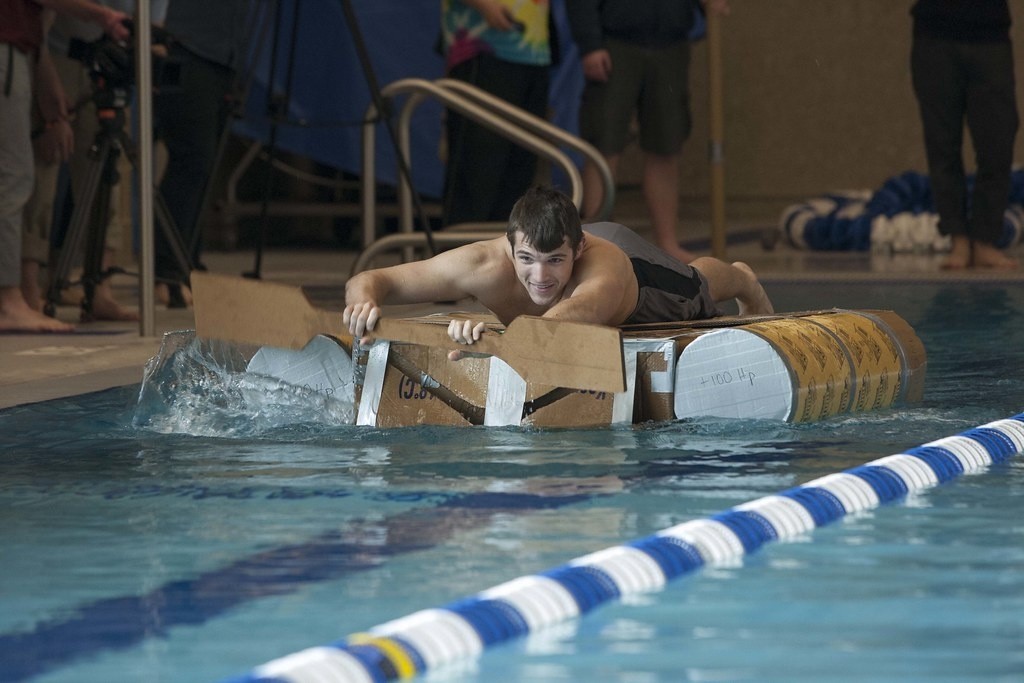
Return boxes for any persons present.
[341,182,775,344]
[0,1,710,333]
[910,0,1023,270]
